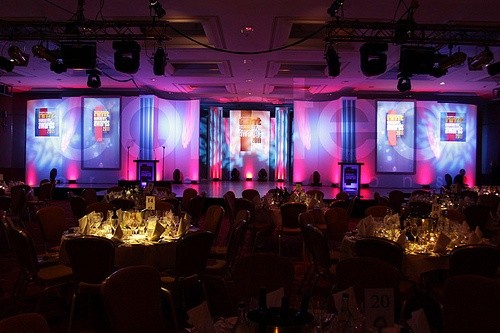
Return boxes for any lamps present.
[0,45,30,72]
[149,0,166,18]
[152,46,167,76]
[112,40,142,74]
[359,40,388,76]
[32,44,101,89]
[397,45,500,92]
[327,0,344,17]
[325,44,341,76]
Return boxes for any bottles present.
[111,207,118,234]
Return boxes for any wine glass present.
[411,227,429,245]
[128,219,146,235]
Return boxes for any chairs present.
[0,167,500,333]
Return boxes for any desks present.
[338,221,495,279]
[60,226,199,273]
[193,310,405,333]
[264,201,327,227]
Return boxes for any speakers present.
[62,44,96,70]
[399,45,435,74]
